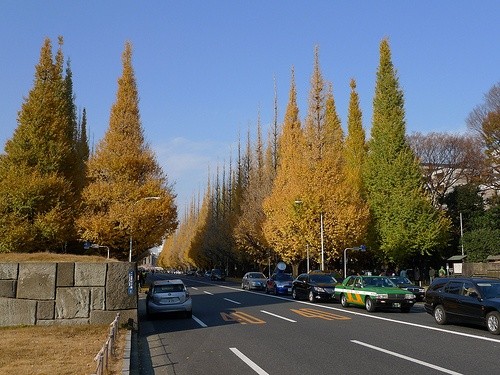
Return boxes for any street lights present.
[294,200,325,271]
[129,196,161,262]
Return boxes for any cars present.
[241,272,268,291]
[291,273,338,303]
[174,270,183,275]
[425,277,500,336]
[188,271,195,276]
[195,271,202,277]
[144,279,193,321]
[374,277,426,299]
[334,276,417,313]
[266,272,296,296]
[205,271,211,278]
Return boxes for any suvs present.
[210,269,227,282]
